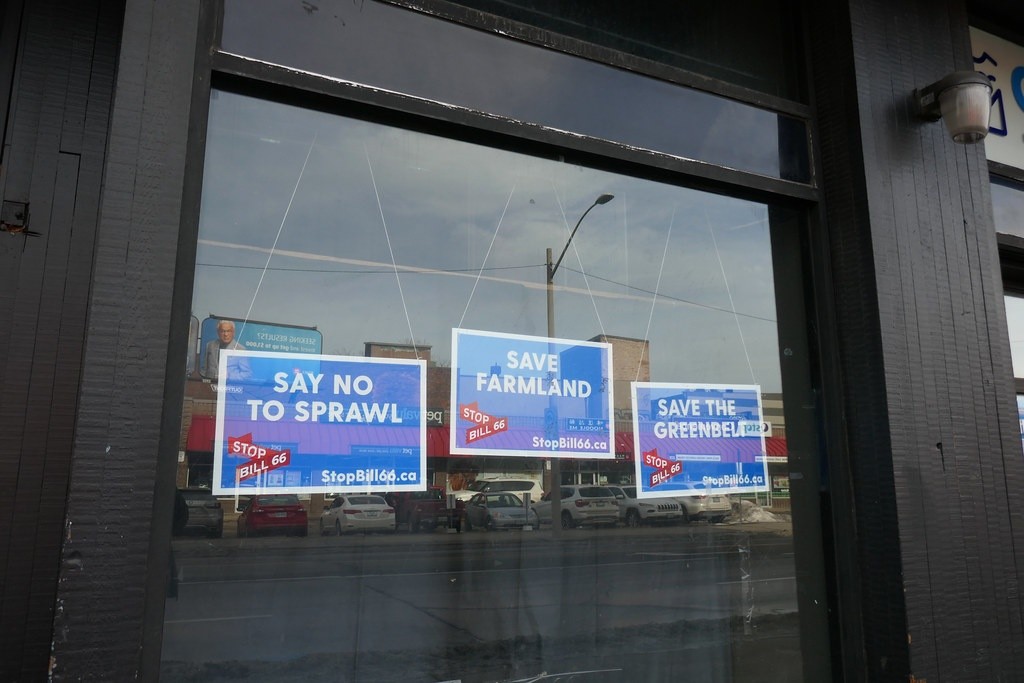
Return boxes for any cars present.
[319,494,397,535]
[679,479,734,523]
[174,486,223,536]
[386,484,465,533]
[464,490,540,531]
[616,485,684,527]
[236,493,308,537]
[530,483,623,527]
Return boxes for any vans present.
[452,478,544,503]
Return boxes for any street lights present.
[542,186,616,528]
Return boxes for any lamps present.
[913,68,993,143]
